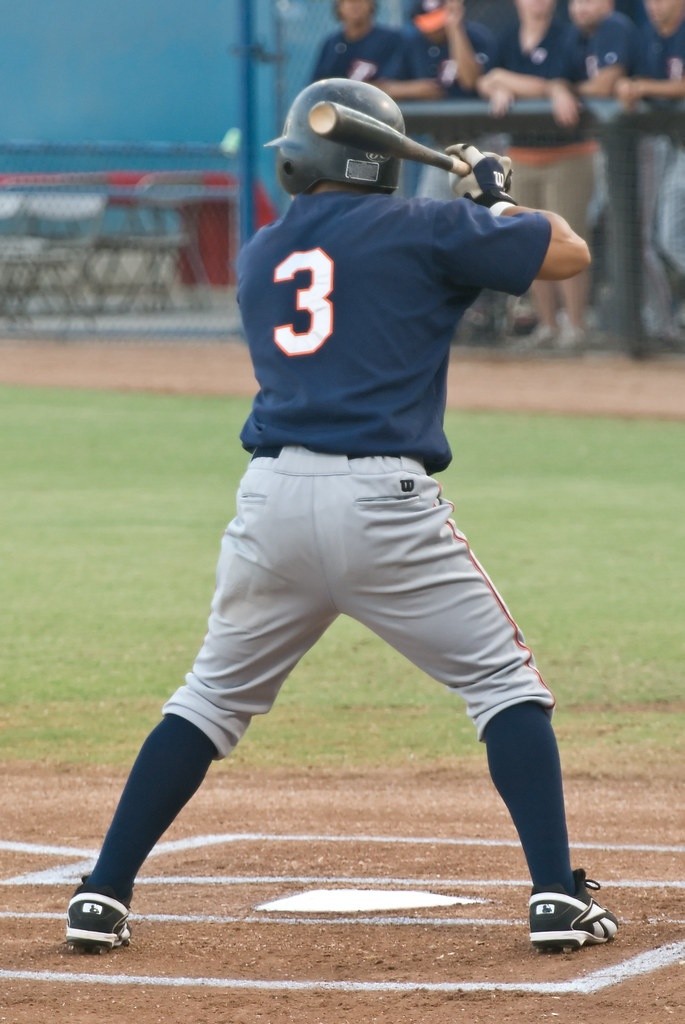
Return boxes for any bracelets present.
[489,202,514,218]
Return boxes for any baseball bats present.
[304,96,514,193]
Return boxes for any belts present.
[247,445,286,460]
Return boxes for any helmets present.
[262,76,406,198]
[411,0,442,22]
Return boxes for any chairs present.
[0,155,224,321]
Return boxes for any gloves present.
[444,142,519,206]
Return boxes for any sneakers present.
[530,870,619,954]
[64,872,134,956]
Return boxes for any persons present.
[307,0,685,366]
[64,78,618,955]
